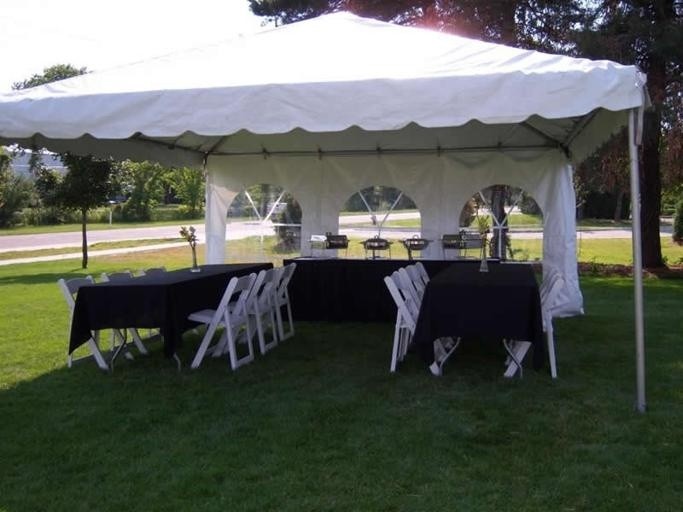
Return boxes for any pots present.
[359,235,394,250]
[397,234,434,249]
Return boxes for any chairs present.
[383,261,453,377]
[58,262,300,371]
[501,260,564,380]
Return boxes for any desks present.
[424,262,541,373]
[282,256,480,325]
